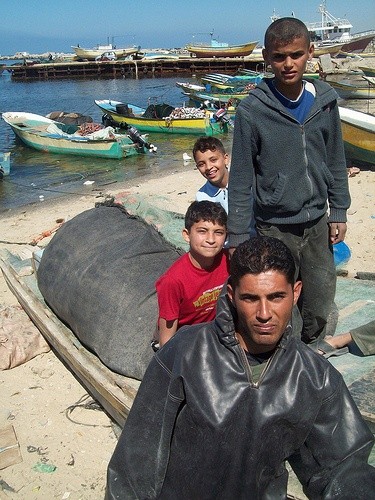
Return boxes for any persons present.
[226,17,351,344]
[105,237,375,500]
[305,318,375,359]
[152,200,230,353]
[193,136,231,216]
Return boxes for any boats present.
[310,43,349,58]
[186,39,260,60]
[237,67,320,80]
[70,37,154,60]
[323,33,375,55]
[2,111,153,160]
[359,66,375,77]
[176,71,267,115]
[94,98,231,137]
[270,0,353,40]
[337,105,375,164]
[323,79,375,99]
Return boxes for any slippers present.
[308,335,349,360]
